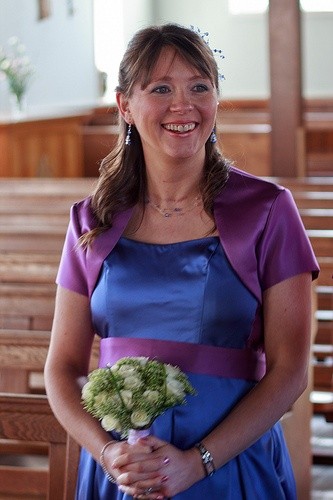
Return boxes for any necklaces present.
[147,194,204,217]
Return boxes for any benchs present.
[0,97,333,500]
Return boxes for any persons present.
[44,22,320,500]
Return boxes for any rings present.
[145,486,152,495]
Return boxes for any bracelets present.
[193,440,215,478]
[100,440,117,484]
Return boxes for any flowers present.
[80,355,198,441]
